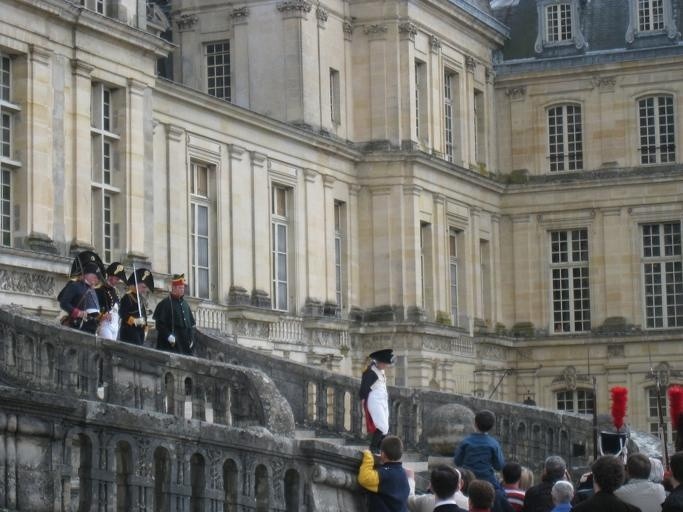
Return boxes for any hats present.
[170,273,185,286]
[105,262,128,285]
[368,349,394,365]
[70,252,107,282]
[600,431,629,460]
[127,267,155,294]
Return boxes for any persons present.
[97,262,129,342]
[358,436,410,511]
[406,450,683,512]
[152,273,197,356]
[57,251,106,335]
[360,349,394,454]
[454,410,506,489]
[119,268,154,346]
